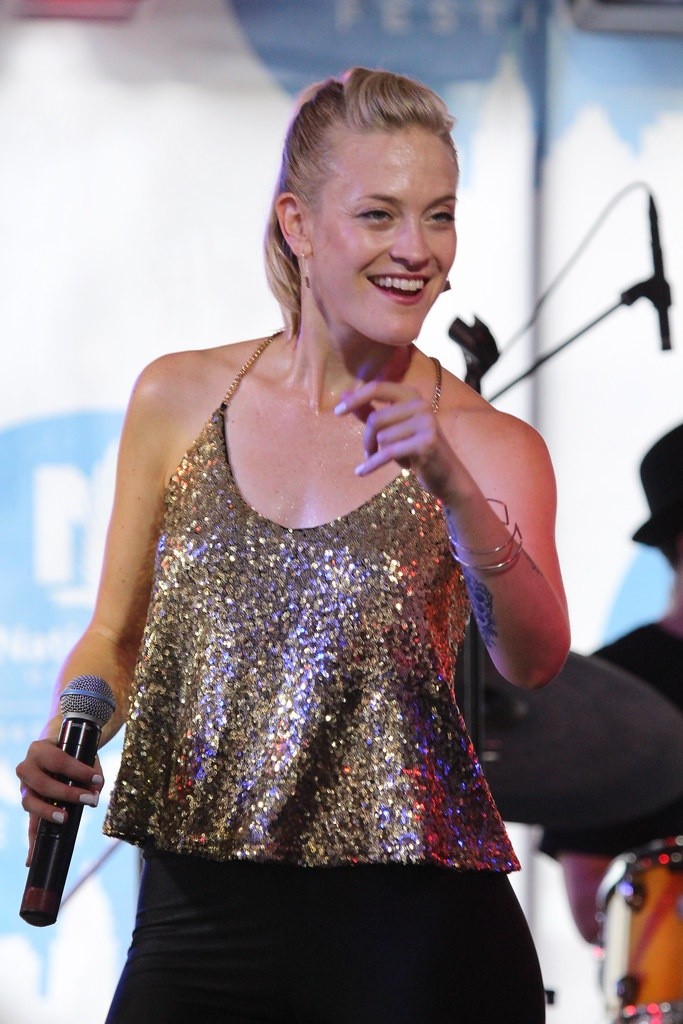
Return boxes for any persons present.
[537,419,683,947]
[14,66,570,1024]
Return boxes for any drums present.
[593,840,683,1024]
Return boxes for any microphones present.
[649,193,671,350]
[20,676,118,927]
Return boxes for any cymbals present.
[449,618,683,832]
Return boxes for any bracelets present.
[448,497,524,570]
